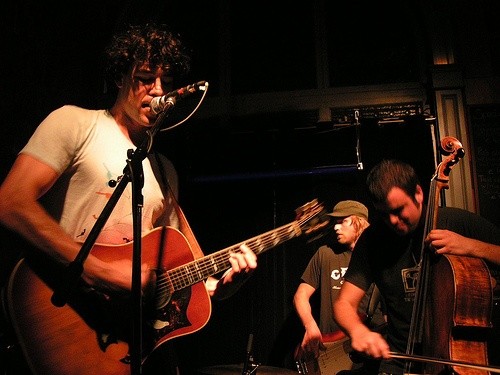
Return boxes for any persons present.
[0,22,257,375]
[335,161,500,375]
[292,199,370,357]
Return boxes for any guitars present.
[7,198,328,375]
[293,313,389,375]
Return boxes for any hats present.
[326,200,368,219]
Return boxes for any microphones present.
[154,81,206,118]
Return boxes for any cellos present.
[401,135,491,375]
